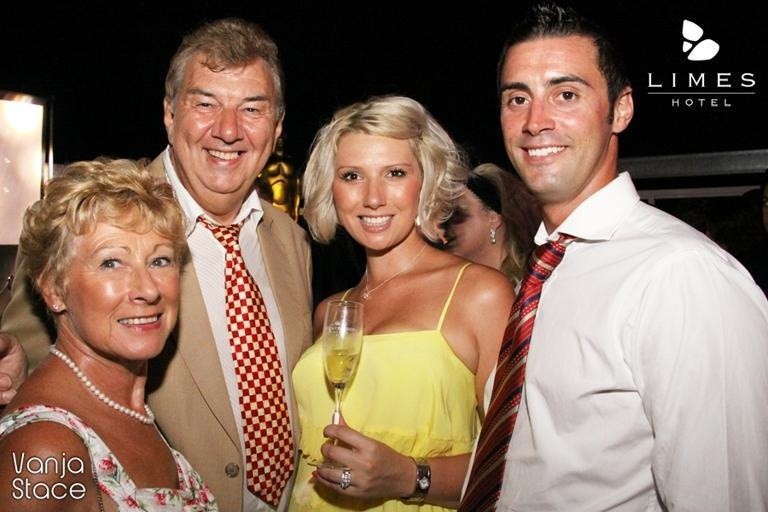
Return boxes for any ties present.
[456,233,577,512]
[197,215,296,511]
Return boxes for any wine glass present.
[305,298,367,471]
